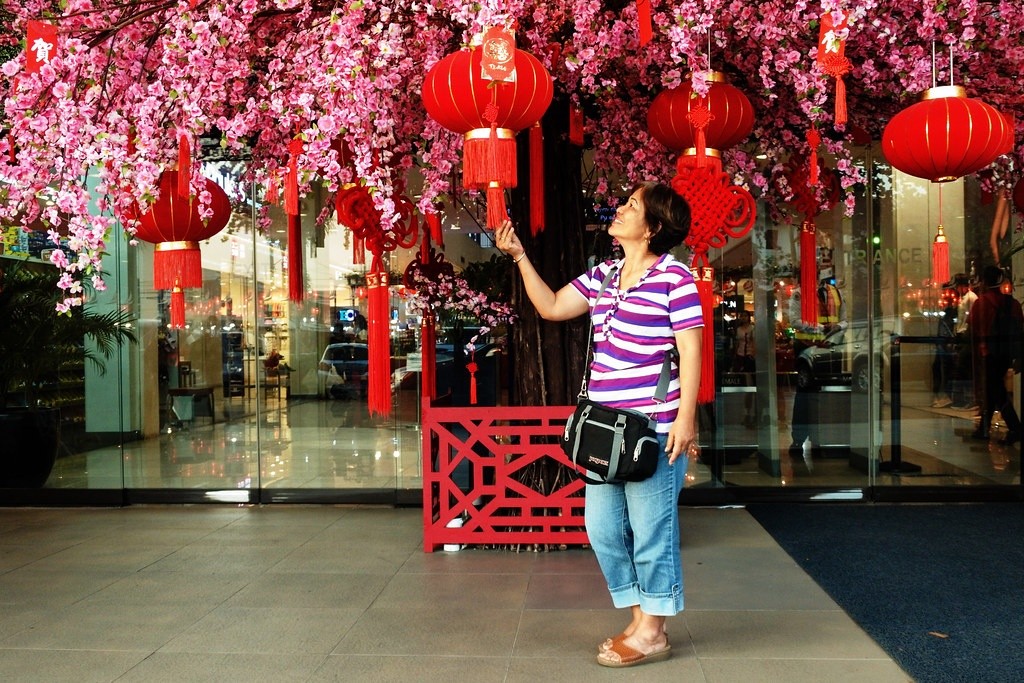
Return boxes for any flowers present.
[0,0,1024,366]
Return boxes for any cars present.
[390,343,501,396]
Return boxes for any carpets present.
[693,444,1024,683]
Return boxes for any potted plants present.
[0,256,142,494]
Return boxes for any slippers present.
[595,624,671,668]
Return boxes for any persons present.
[969,266,1024,444]
[496,183,705,665]
[788,261,848,456]
[947,273,980,411]
[926,281,959,409]
[734,311,755,408]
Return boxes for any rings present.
[501,237,506,240]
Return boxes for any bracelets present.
[513,251,525,263]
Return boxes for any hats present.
[979,266,1003,286]
[942,274,968,289]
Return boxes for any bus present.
[442,326,487,344]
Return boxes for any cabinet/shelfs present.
[264,300,289,353]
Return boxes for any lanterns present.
[421,43,554,230]
[882,85,1014,283]
[646,69,755,189]
[121,172,231,329]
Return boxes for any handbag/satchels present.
[560,396,658,484]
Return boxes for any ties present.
[819,286,826,301]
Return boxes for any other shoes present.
[931,396,978,412]
[971,427,990,441]
[998,430,1023,445]
[788,440,802,454]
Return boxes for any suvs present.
[319,342,369,400]
[794,314,959,395]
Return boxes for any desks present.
[264,368,297,403]
[171,384,216,427]
